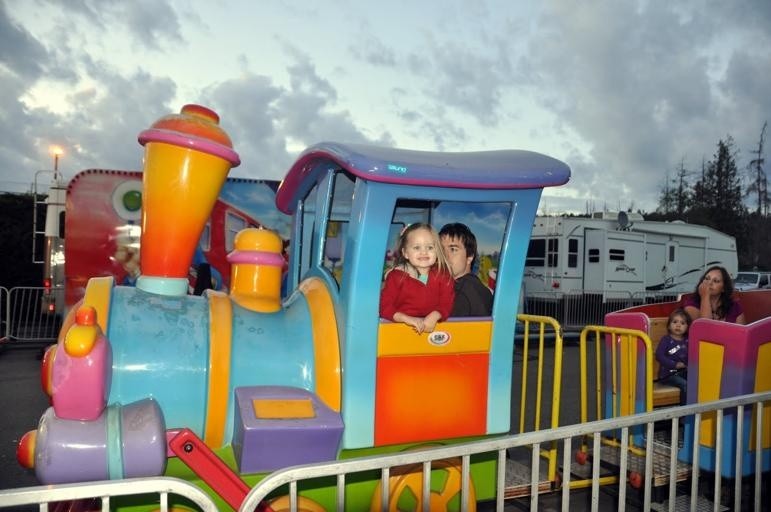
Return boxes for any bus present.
[32,170,67,337]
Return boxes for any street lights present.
[50,144,65,180]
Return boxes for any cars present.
[733,272,771,291]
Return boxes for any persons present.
[657,307,694,406]
[682,265,747,327]
[381,220,455,334]
[439,223,496,316]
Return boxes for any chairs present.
[646,317,681,405]
